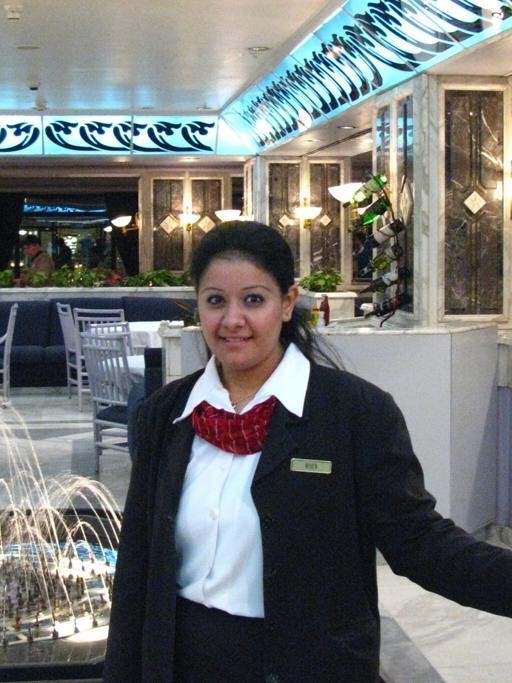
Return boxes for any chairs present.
[54,301,149,473]
[0,303,19,401]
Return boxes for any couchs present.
[0,296,197,386]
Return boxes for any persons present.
[102,221,512,683]
[352,231,373,279]
[23,235,53,272]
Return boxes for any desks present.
[87,319,185,354]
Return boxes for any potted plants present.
[294,266,358,323]
